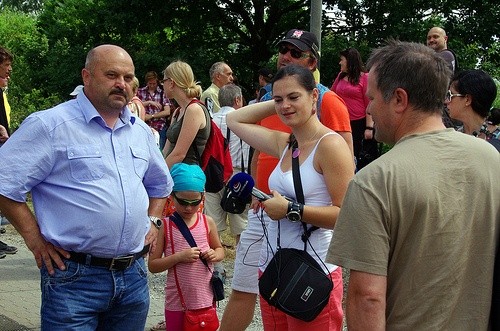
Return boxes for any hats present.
[170,162,206,193]
[275,29,319,59]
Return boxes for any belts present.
[58,245,150,272]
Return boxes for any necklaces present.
[292,127,320,158]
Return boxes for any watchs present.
[286,203,301,223]
[149,216,162,229]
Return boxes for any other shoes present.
[0,250,6,258]
[4,246,18,254]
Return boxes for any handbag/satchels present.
[211,267,226,302]
[260,246,334,323]
[182,307,220,331]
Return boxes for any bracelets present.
[365,126,373,130]
[152,114,154,118]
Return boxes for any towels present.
[260,83,329,120]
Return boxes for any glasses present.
[278,45,309,58]
[444,90,462,102]
[158,78,169,85]
[174,193,202,206]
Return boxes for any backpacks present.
[173,97,233,193]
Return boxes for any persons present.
[225,63,355,331]
[0,45,17,258]
[125,76,146,124]
[0,44,175,331]
[203,84,249,276]
[161,61,211,214]
[325,36,500,331]
[148,162,226,331]
[444,68,500,153]
[199,61,233,116]
[232,69,273,174]
[134,70,170,150]
[330,46,379,173]
[427,27,455,78]
[219,30,356,331]
